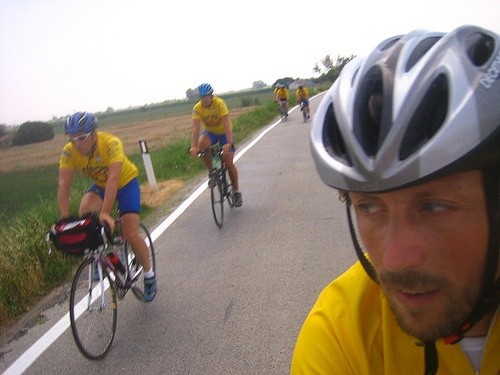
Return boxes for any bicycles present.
[46,213,156,361]
[278,100,288,122]
[298,99,309,123]
[187,148,235,228]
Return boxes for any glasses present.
[69,131,92,143]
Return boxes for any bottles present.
[107,252,126,274]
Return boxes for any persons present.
[289,24,499,375]
[58,111,157,302]
[189,84,242,208]
[273,83,289,116]
[296,86,310,119]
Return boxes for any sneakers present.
[91,264,101,280]
[143,276,157,301]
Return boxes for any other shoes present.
[231,190,243,207]
[208,170,216,188]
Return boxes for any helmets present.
[198,83,214,96]
[279,85,285,88]
[309,24,500,192]
[276,84,280,87]
[64,112,98,135]
